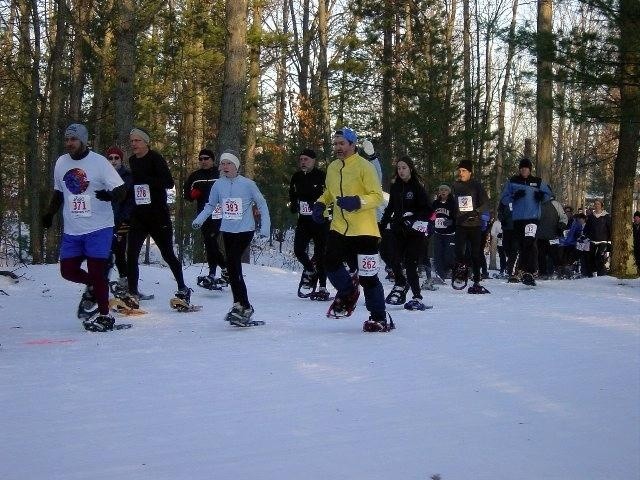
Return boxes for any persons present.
[497,159,555,286]
[38,123,127,331]
[428,159,491,292]
[491,195,640,280]
[312,127,390,330]
[191,146,272,325]
[102,144,136,294]
[111,126,192,312]
[183,146,232,286]
[378,154,435,311]
[289,147,335,298]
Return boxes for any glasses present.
[107,154,121,162]
[198,156,210,162]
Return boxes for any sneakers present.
[202,273,214,287]
[78,281,140,331]
[496,270,586,286]
[175,287,191,311]
[217,268,229,285]
[300,264,425,332]
[235,304,253,325]
[420,267,489,293]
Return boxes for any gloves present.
[290,202,300,216]
[513,190,525,200]
[444,219,453,226]
[466,210,478,222]
[403,218,416,231]
[312,204,326,221]
[336,196,360,212]
[94,188,113,202]
[535,191,545,203]
[41,212,53,228]
[363,140,375,156]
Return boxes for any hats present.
[105,147,124,163]
[335,129,357,145]
[299,150,316,163]
[219,150,240,172]
[66,124,88,147]
[459,160,472,172]
[129,126,152,146]
[198,149,216,161]
[518,159,533,171]
